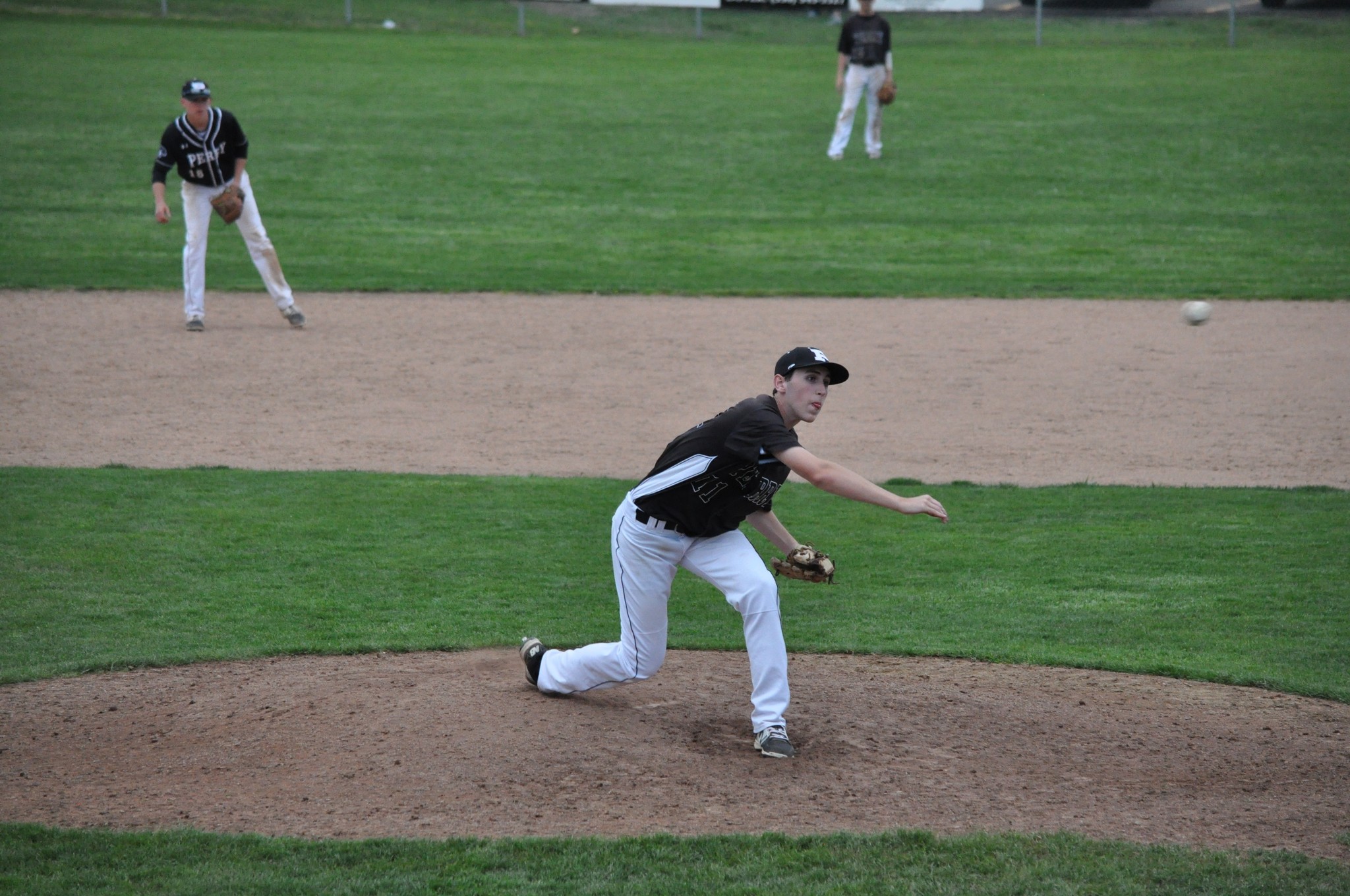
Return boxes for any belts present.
[635,508,688,534]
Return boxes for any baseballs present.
[1180,299,1214,324]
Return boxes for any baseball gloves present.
[878,80,897,104]
[211,185,245,225]
[770,545,835,584]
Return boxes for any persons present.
[826,0,898,161]
[519,347,947,760]
[151,80,305,329]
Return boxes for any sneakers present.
[520,638,546,684]
[755,725,795,759]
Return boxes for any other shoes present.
[186,321,203,331]
[289,312,307,326]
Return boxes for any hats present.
[775,346,849,385]
[182,79,210,101]
